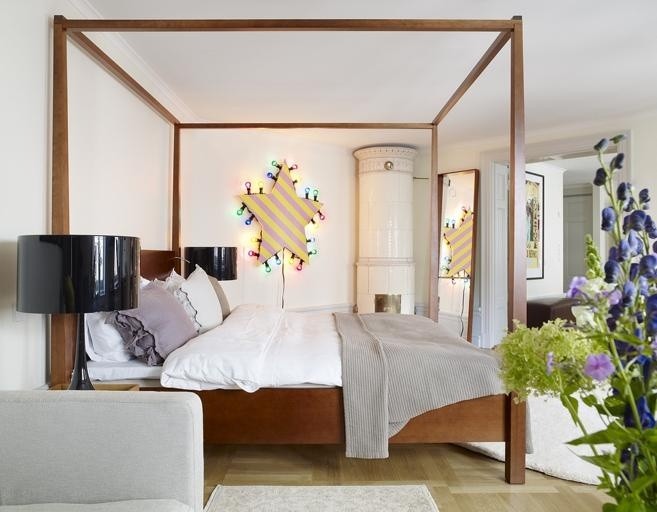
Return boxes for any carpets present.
[453,387,621,487]
[204,484,440,512]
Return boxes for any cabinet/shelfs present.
[527,298,589,328]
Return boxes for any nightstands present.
[46,383,139,392]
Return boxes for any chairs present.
[0,391,204,512]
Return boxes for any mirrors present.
[435,169,479,342]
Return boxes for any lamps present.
[184,247,237,280]
[16,235,140,390]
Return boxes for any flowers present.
[503,141,657,512]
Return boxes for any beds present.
[50,14,527,485]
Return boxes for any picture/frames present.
[525,170,544,280]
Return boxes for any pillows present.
[83,264,231,366]
